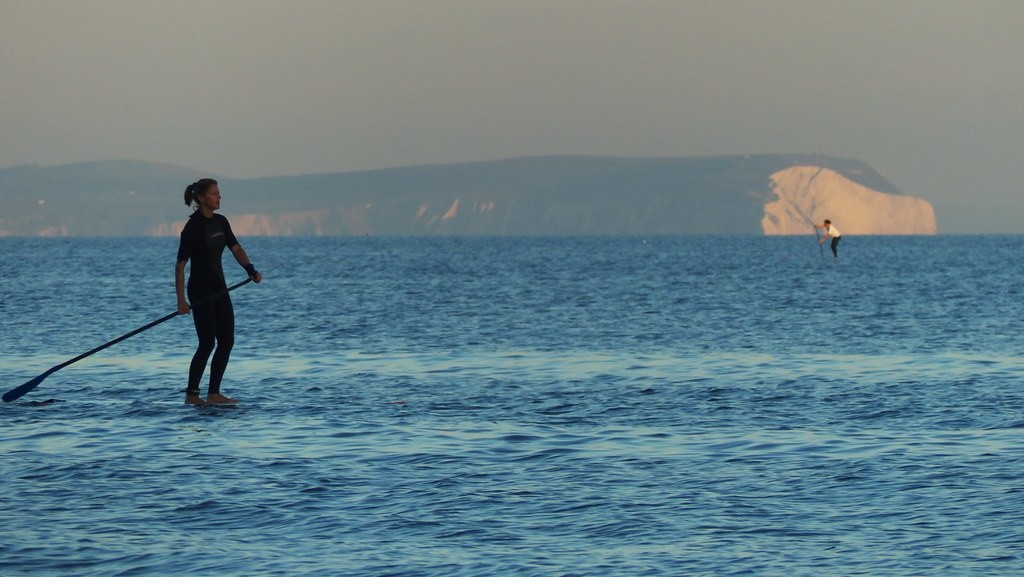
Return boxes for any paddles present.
[1,276,252,405]
[814,226,824,257]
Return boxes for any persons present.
[175,178,262,404]
[814,219,842,257]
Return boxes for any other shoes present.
[207,393,236,405]
[184,394,206,405]
